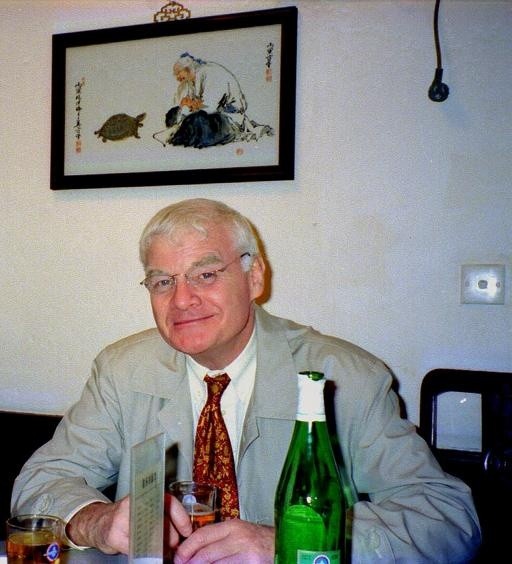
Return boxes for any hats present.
[191,373,240,523]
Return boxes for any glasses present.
[140,251,252,295]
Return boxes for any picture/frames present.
[50,1,295,188]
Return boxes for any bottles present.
[273,374,357,564]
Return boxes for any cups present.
[3,516,62,562]
[166,480,218,536]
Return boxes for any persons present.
[164,50,247,149]
[7,193,488,564]
